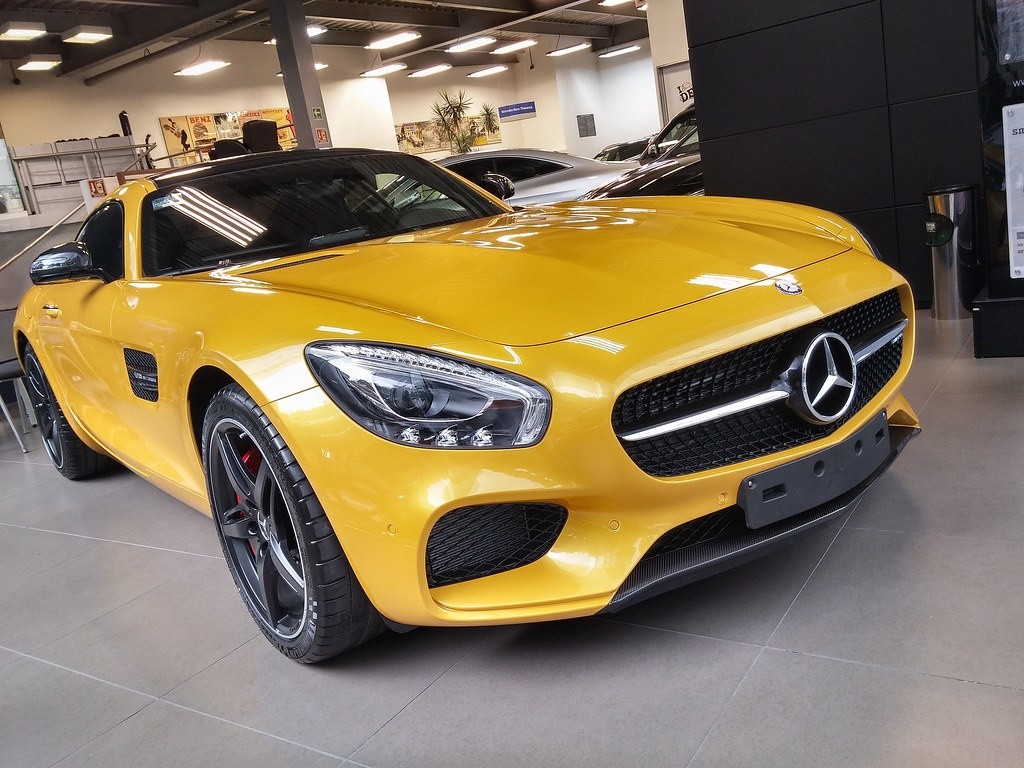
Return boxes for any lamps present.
[598,0,632,6]
[546,42,591,56]
[173,61,232,75]
[61,25,112,44]
[17,54,62,70]
[598,44,642,58]
[360,63,408,77]
[365,31,422,49]
[636,3,647,11]
[408,62,452,77]
[0,21,47,41]
[488,40,539,55]
[445,36,497,53]
[276,64,328,76]
[264,24,328,45]
[467,65,509,77]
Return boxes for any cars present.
[583,102,700,201]
[350,149,644,232]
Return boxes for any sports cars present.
[12,148,922,664]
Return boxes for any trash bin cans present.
[925,183,977,320]
